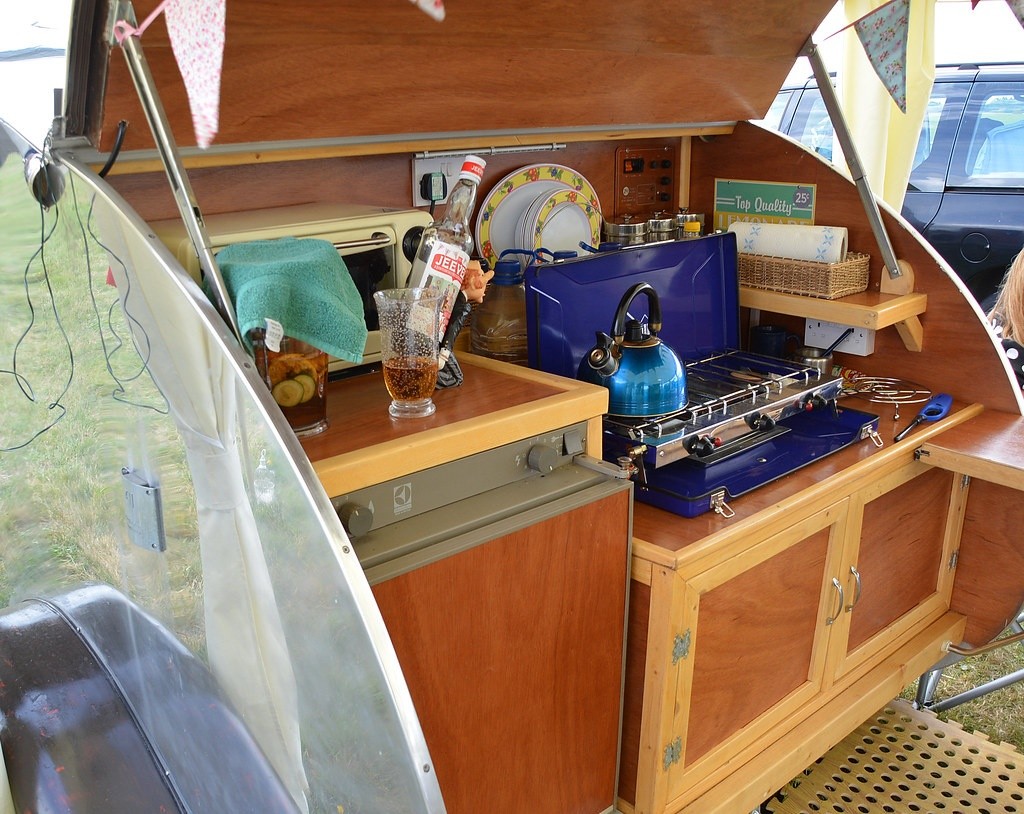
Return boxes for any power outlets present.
[412,159,465,207]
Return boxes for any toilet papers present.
[727,221,849,265]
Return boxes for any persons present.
[987,247,1024,396]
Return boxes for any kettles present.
[578,280,688,419]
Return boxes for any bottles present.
[679,222,701,241]
[470,240,622,368]
[394,155,487,357]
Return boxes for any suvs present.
[749,61,1023,310]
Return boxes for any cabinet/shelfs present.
[617,446,972,814]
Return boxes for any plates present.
[480,162,604,265]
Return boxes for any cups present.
[247,325,330,439]
[795,345,834,375]
[750,324,801,359]
[374,288,443,418]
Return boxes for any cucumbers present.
[272,374,315,407]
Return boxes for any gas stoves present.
[602,349,839,486]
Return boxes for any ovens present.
[146,202,439,374]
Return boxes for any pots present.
[602,206,706,246]
